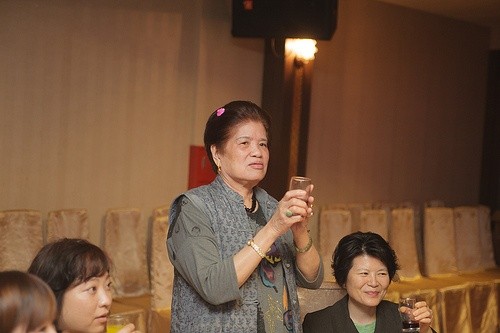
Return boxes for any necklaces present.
[245,194,256,212]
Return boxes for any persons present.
[0,270,57,333]
[167,101,324,333]
[303,231,439,333]
[27,238,135,333]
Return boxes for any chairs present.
[0,201,500,333]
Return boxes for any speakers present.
[230,0,339,41]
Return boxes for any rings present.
[310,204,314,216]
[286,210,292,216]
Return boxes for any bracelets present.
[246,239,266,258]
[294,237,312,252]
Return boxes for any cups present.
[106,315,129,333]
[398,297,420,332]
[289,176,312,195]
